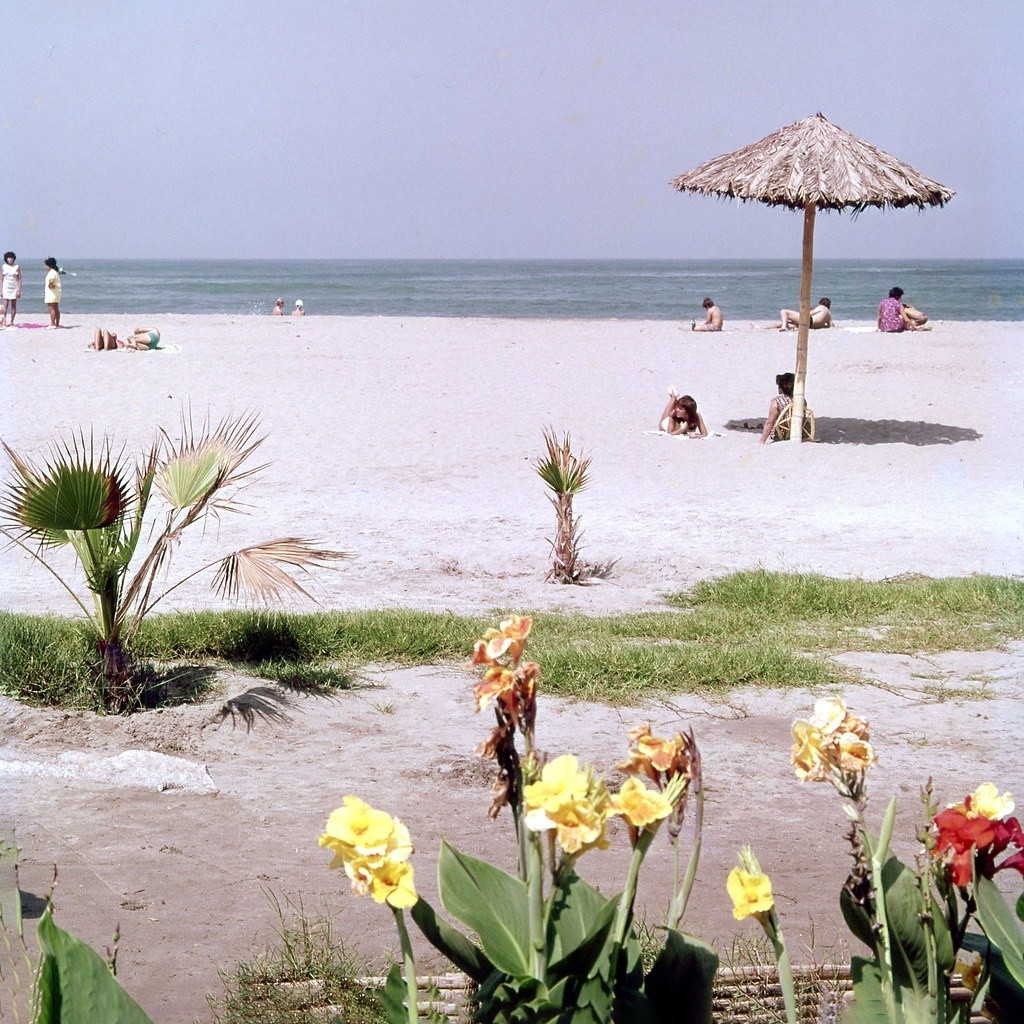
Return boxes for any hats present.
[296,299,303,307]
[776,373,795,393]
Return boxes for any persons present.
[876,286,926,335]
[91,327,161,350]
[272,298,305,316]
[757,373,807,445]
[659,384,708,439]
[692,287,727,332]
[0,252,61,329]
[780,298,834,329]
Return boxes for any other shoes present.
[46,326,56,330]
